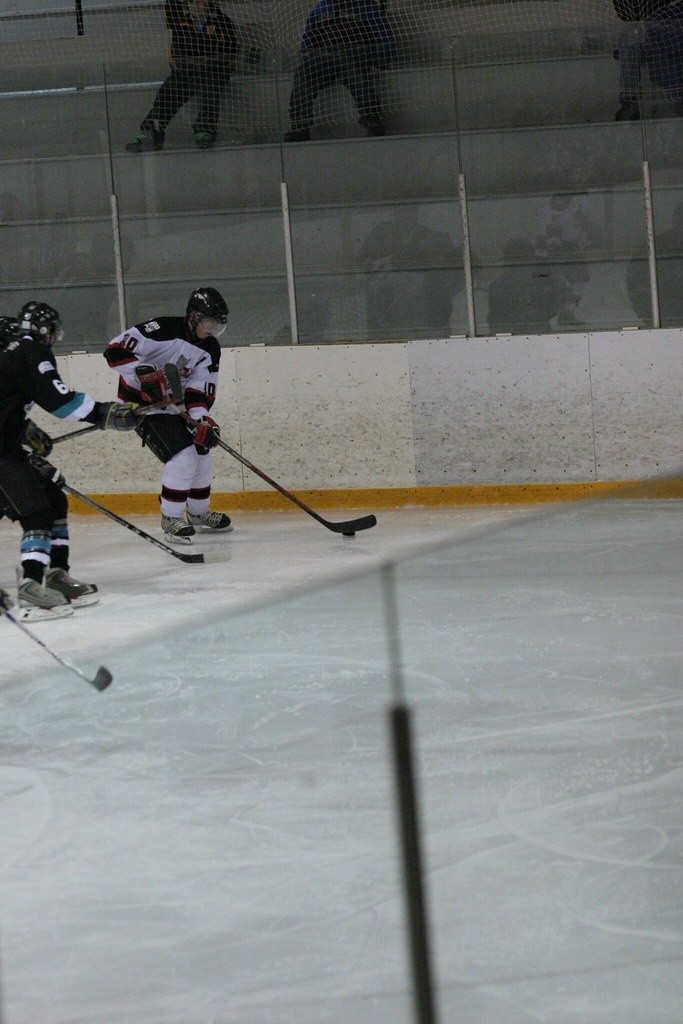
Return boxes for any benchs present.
[0,0,683,347]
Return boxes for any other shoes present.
[366,122,385,137]
[615,100,640,121]
[283,129,310,142]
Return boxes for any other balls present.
[342,530,355,536]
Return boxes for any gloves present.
[0,589,14,617]
[20,418,53,458]
[134,364,168,403]
[186,415,220,448]
[99,402,144,431]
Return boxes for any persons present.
[283,0,395,143]
[613,0,683,121]
[102,287,235,546]
[0,301,144,622]
[124,0,237,152]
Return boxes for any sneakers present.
[124,127,162,153]
[161,512,196,545]
[194,132,215,149]
[15,565,75,622]
[186,511,233,533]
[45,567,99,608]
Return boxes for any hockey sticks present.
[178,407,376,533]
[40,360,186,446]
[60,481,231,563]
[1,604,114,691]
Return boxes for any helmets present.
[16,300,62,349]
[186,286,229,326]
